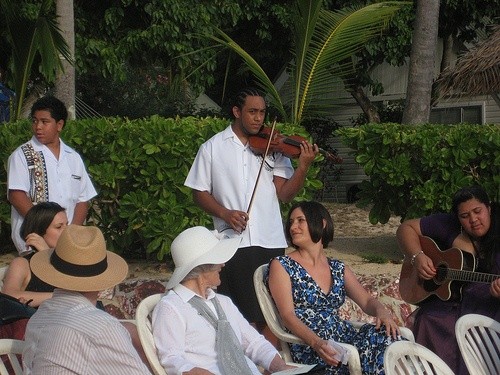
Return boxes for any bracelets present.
[411,251,423,264]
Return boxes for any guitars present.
[398,236,500,308]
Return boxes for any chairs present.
[253,264,415,375]
[455,314,500,375]
[383,340,455,375]
[137,293,166,375]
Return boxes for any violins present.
[250,126,343,165]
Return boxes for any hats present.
[165,226,243,290]
[30,224,128,292]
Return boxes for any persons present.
[0,202,69,307]
[7,95,98,257]
[152,226,326,375]
[396,187,500,375]
[21,224,154,375]
[262,200,410,375]
[184,87,319,334]
